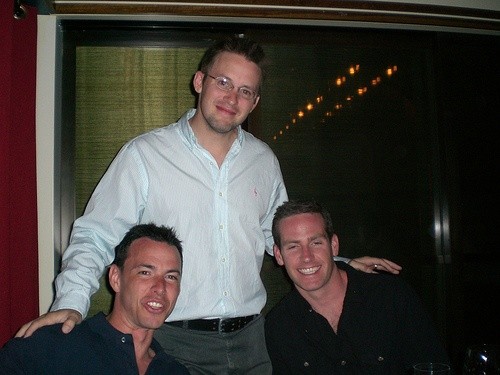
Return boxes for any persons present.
[264,201,448,375]
[0,222,191,375]
[15,39,401,375]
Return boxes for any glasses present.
[203,71,258,100]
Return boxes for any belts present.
[165,314,258,335]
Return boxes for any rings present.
[373,264,378,270]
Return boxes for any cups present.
[463,344,500,375]
[414,362,451,375]
